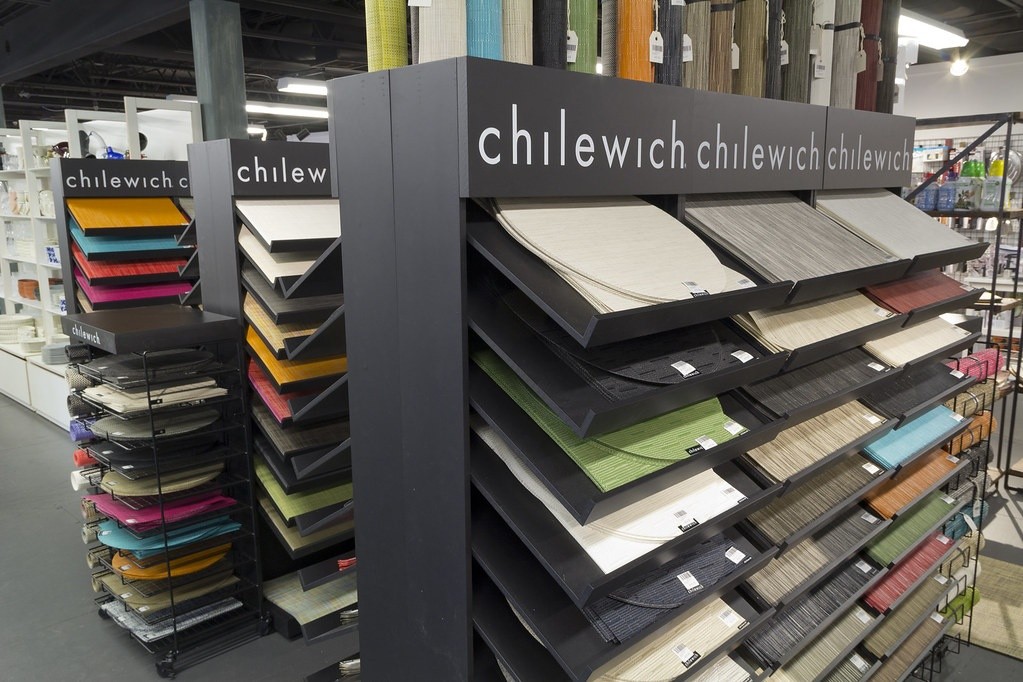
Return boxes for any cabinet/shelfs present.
[0,56,1023,682]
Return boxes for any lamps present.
[297,126,310,140]
[951,49,970,77]
[164,93,330,120]
[277,76,328,96]
[897,7,969,50]
